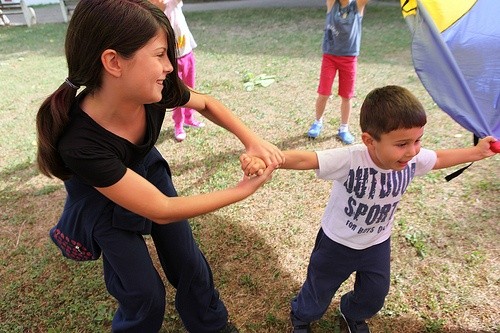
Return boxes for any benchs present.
[0,0,36,29]
[60,0,80,23]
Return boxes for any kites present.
[400,0,499,153]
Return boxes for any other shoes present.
[338,128,355,143]
[290,322,312,333]
[307,122,324,136]
[175,123,186,140]
[339,301,371,333]
[184,118,205,127]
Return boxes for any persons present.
[241,85,500,333]
[36,0,286,333]
[148,0,205,141]
[307,0,368,144]
